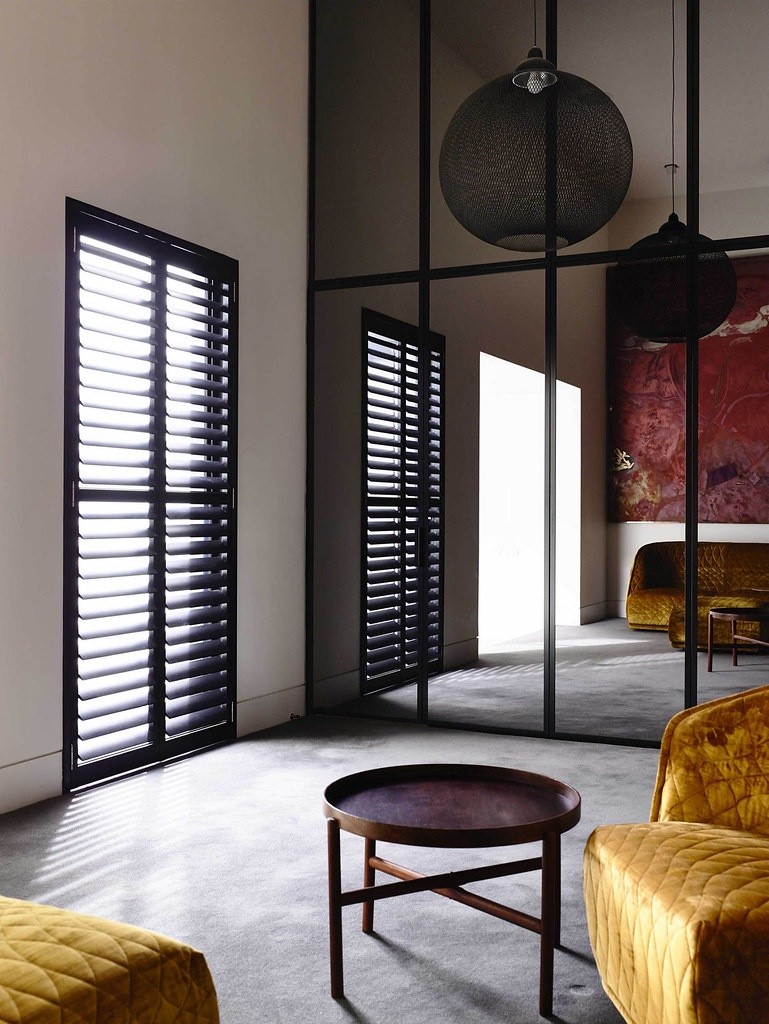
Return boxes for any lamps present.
[657,0,687,246]
[511,0,558,94]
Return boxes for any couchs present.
[0,896,220,1024]
[626,542,769,654]
[582,684,769,1024]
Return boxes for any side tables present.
[322,762,581,1018]
[707,608,769,672]
